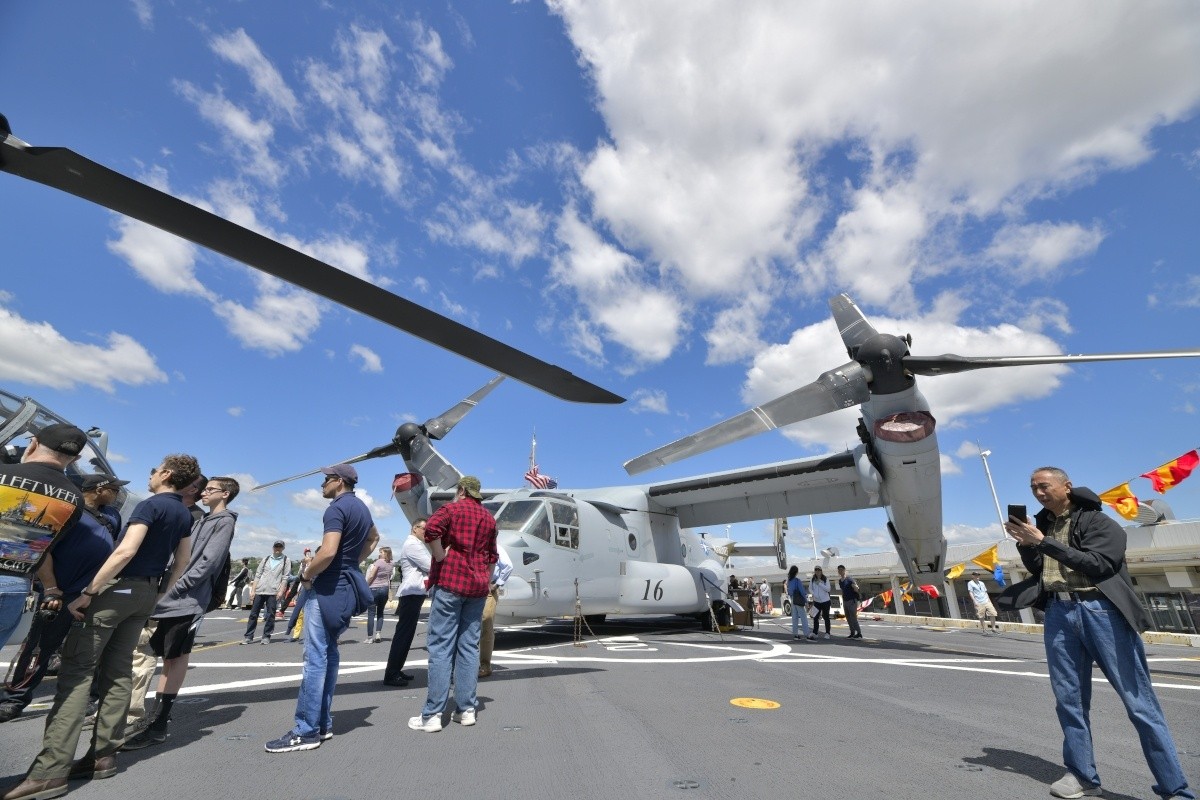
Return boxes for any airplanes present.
[1,112,1198,694]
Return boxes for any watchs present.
[81,587,98,596]
[299,573,310,582]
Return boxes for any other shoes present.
[845,634,855,640]
[375,634,381,643]
[260,637,270,645]
[121,730,167,750]
[479,668,490,677]
[794,635,802,641]
[852,634,862,640]
[240,639,254,645]
[364,638,373,644]
[81,714,96,731]
[134,713,172,730]
[384,671,415,686]
[824,633,830,640]
[806,636,815,642]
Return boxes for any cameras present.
[34,603,60,623]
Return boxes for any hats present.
[274,540,285,548]
[458,476,484,500]
[82,474,131,491]
[24,424,87,455]
[319,465,359,486]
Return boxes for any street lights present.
[979,448,1007,538]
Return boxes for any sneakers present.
[265,731,321,754]
[1050,773,1102,798]
[449,708,476,726]
[0,778,71,799]
[408,712,443,732]
[318,727,333,740]
[71,753,116,780]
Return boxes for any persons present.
[276,545,321,642]
[225,558,249,610]
[477,544,514,678]
[362,547,394,644]
[809,565,832,638]
[1000,467,1200,800]
[967,572,1000,634]
[838,565,862,640]
[383,519,431,686]
[0,454,201,800]
[0,424,87,651]
[264,463,379,752]
[786,566,819,643]
[0,475,130,721]
[728,575,770,614]
[117,474,241,750]
[241,540,291,645]
[408,477,499,732]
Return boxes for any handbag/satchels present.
[793,578,806,607]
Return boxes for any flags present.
[856,582,915,608]
[525,464,557,489]
[917,585,940,599]
[1139,450,1200,495]
[944,545,1006,588]
[1096,483,1138,520]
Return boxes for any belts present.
[1043,589,1108,601]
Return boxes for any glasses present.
[150,468,160,476]
[200,486,225,494]
[105,486,120,493]
[325,476,337,483]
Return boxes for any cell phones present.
[1008,502,1027,525]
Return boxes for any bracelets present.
[44,586,57,592]
